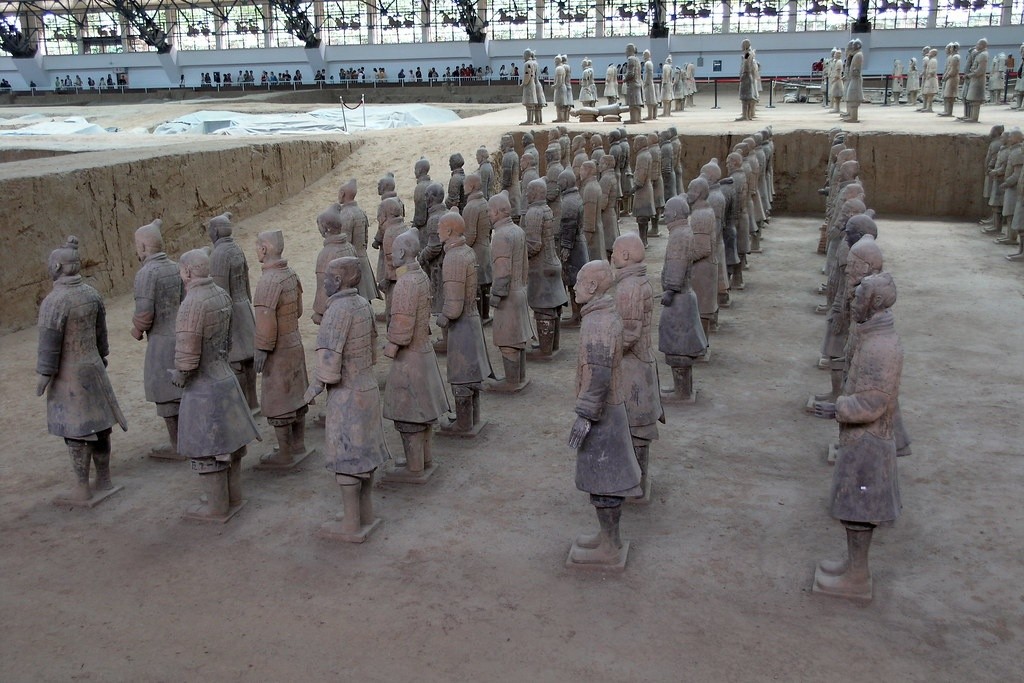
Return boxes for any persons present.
[735,39,763,122]
[55,74,127,90]
[813,59,847,77]
[1006,54,1014,73]
[0,78,13,95]
[979,125,1024,262]
[566,126,774,570]
[521,44,697,126]
[807,128,913,601]
[201,62,663,88]
[132,127,687,545]
[179,74,186,88]
[30,81,37,96]
[891,38,1024,124]
[34,234,128,508]
[821,38,864,123]
